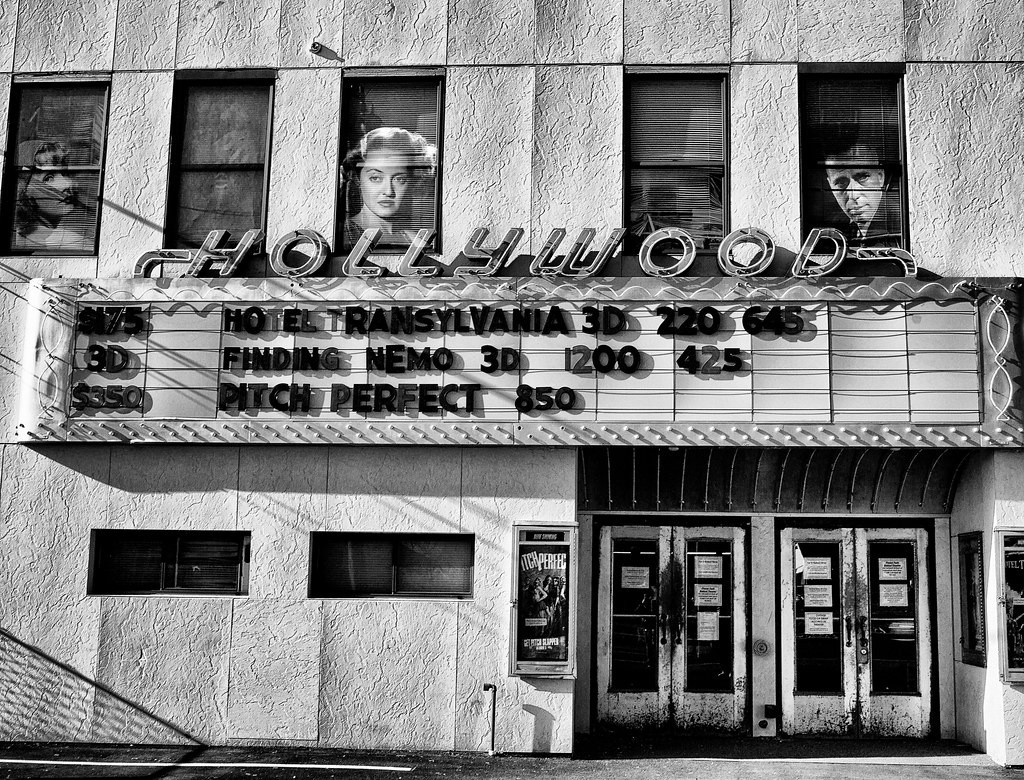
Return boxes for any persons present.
[823,141,903,240]
[12,140,93,250]
[338,125,436,244]
